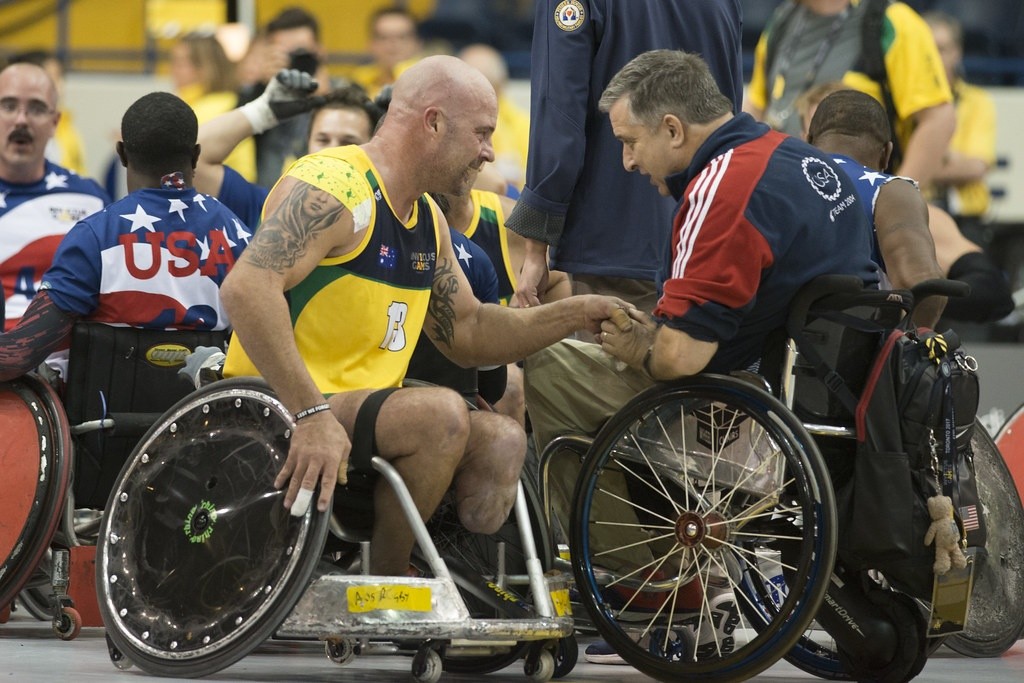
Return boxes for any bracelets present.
[288,402,332,424]
[644,344,656,380]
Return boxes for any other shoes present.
[540,569,705,624]
[585,642,629,665]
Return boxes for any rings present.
[602,332,607,341]
[524,304,529,309]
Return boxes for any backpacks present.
[855,324,990,634]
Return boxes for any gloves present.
[245,68,326,134]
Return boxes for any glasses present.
[2,100,57,117]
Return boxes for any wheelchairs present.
[0,278,1022,682]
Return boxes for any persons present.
[501,1,745,346]
[745,0,1016,329]
[520,48,884,665]
[0,60,113,394]
[219,52,644,581]
[191,2,575,455]
[0,90,252,524]
[799,84,951,347]
